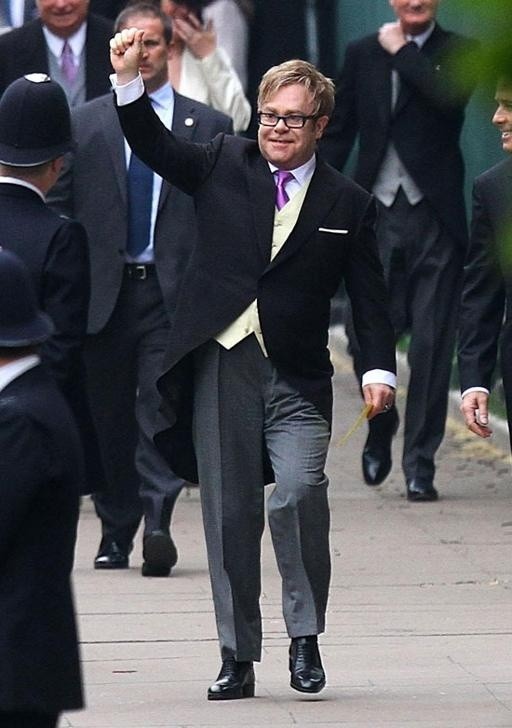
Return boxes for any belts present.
[125,262,156,280]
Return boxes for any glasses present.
[255,111,320,128]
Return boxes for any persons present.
[456,64,511,456]
[316,1,482,503]
[107,26,398,700]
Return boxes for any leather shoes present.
[287,634,326,695]
[206,656,257,701]
[140,538,180,580]
[361,405,401,486]
[92,536,130,570]
[403,478,437,503]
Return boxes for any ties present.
[62,42,79,90]
[271,169,296,211]
[125,151,155,258]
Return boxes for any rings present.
[383,405,391,412]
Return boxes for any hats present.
[0,72,80,169]
